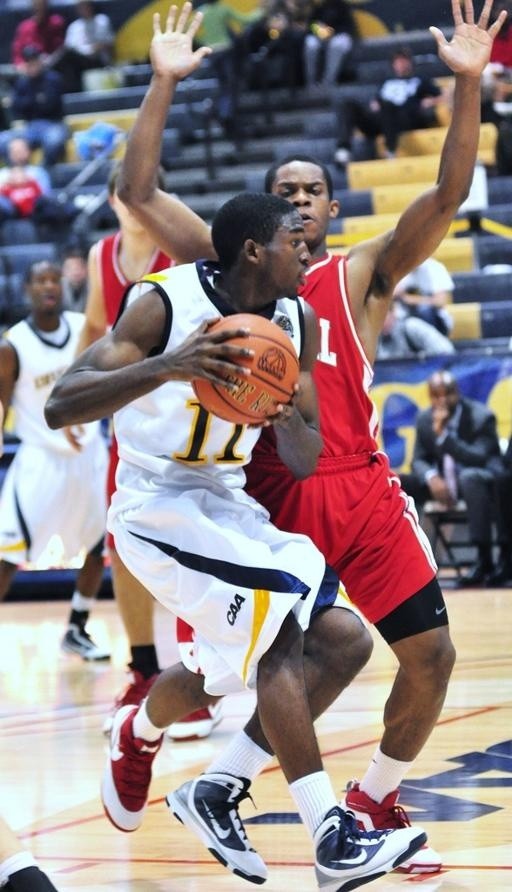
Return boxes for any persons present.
[40,190,433,890]
[93,0,512,882]
[63,160,225,738]
[0,260,110,658]
[0,1,511,588]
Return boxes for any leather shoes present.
[460,564,511,586]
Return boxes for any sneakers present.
[312,781,442,892]
[163,768,269,885]
[102,663,224,831]
[63,622,112,662]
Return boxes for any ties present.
[441,420,459,501]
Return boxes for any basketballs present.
[191,312,298,424]
